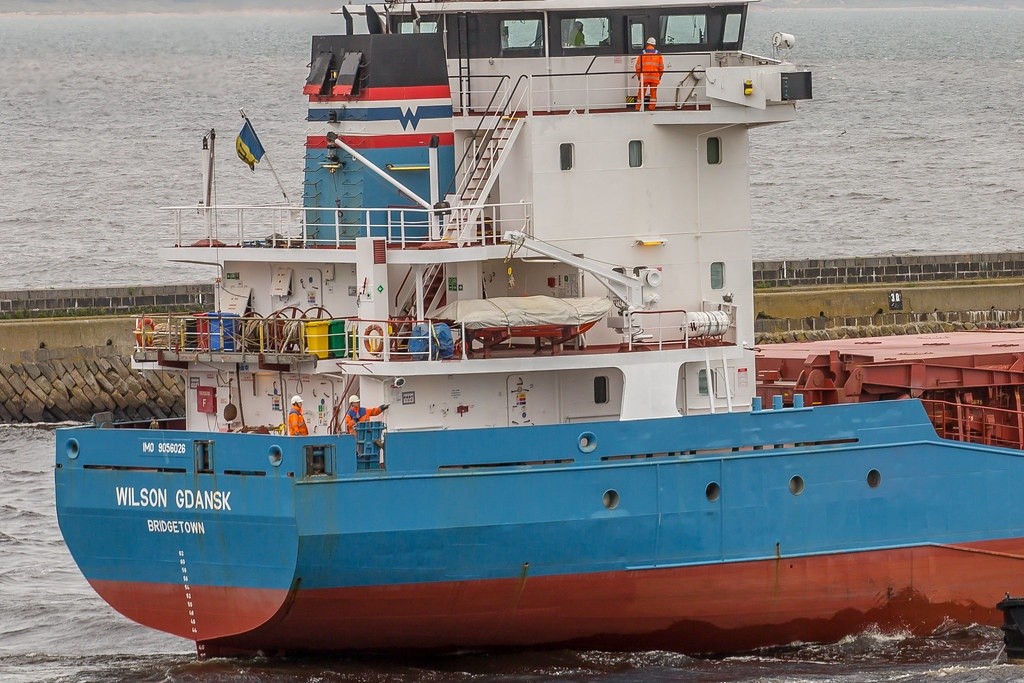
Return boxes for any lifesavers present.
[364,327,383,354]
[136,318,155,346]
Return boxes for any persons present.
[287,396,310,436]
[345,394,391,435]
[569,20,586,46]
[634,37,665,111]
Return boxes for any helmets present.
[647,37,656,45]
[349,395,360,404]
[291,395,303,404]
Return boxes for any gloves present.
[381,404,390,409]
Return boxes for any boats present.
[51,2,1024,657]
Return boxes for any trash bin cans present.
[304,320,346,360]
[185,312,241,354]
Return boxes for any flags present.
[234,120,266,171]
